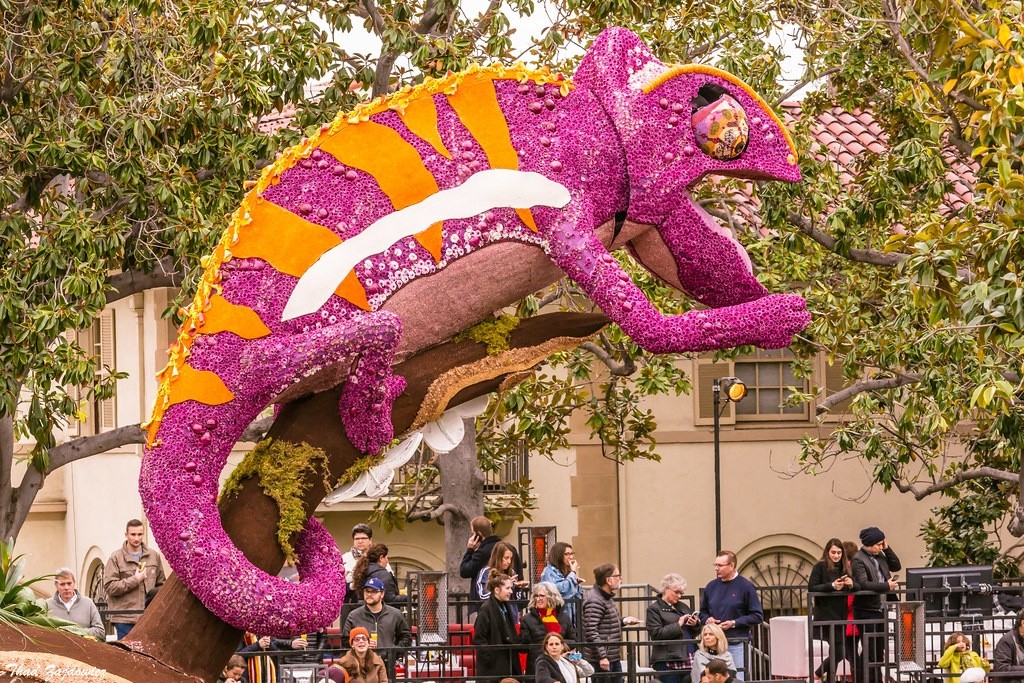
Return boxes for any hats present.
[362,578,384,590]
[860,527,886,546]
[319,663,349,683]
[961,667,987,683]
[350,627,370,646]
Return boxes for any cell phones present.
[685,611,699,624]
[892,575,899,581]
[838,574,847,582]
[474,533,483,543]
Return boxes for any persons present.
[341,523,396,590]
[340,578,412,683]
[103,519,167,640]
[336,626,388,683]
[216,654,247,683]
[519,581,576,683]
[472,568,530,683]
[561,642,595,678]
[476,541,530,636]
[44,567,106,642]
[541,541,586,649]
[536,632,595,683]
[318,655,360,683]
[690,526,1024,683]
[646,573,702,683]
[582,563,640,682]
[235,631,308,683]
[352,543,407,602]
[459,516,524,617]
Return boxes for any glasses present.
[564,552,576,557]
[353,537,370,540]
[713,561,734,567]
[666,587,684,595]
[364,590,382,593]
[609,574,622,577]
[352,636,368,641]
[534,595,546,600]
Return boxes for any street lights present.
[712,375,747,559]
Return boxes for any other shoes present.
[806,677,823,683]
